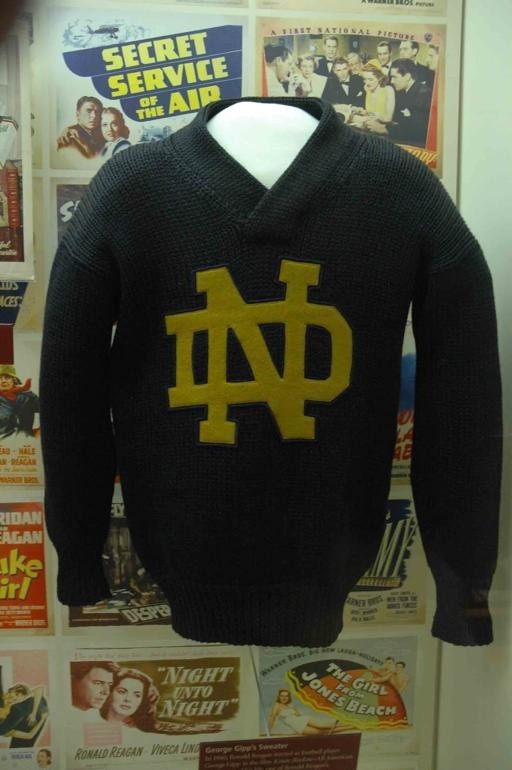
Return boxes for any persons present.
[368,655,410,695]
[100,667,159,733]
[70,661,120,729]
[0,683,53,770]
[264,35,439,149]
[267,689,339,736]
[57,96,105,159]
[56,107,132,158]
[0,364,40,439]
